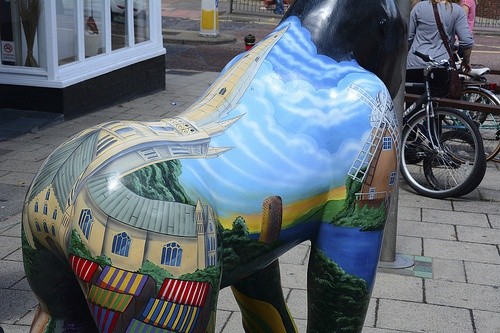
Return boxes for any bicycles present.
[442,64,500,163]
[399,51,484,199]
[403,73,491,199]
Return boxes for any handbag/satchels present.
[450,63,461,100]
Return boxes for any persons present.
[452,0,479,61]
[19,0,411,331]
[403,0,477,126]
